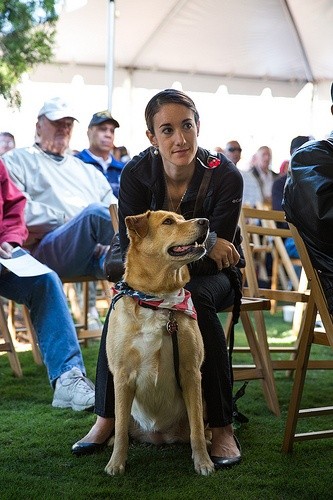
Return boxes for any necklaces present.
[164,170,188,213]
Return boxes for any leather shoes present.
[69,427,114,454]
[209,434,242,467]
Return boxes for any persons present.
[0,132,16,156]
[0,98,118,284]
[289,81,333,316]
[71,86,246,467]
[0,154,97,410]
[68,107,130,198]
[219,133,315,290]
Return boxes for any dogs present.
[99,206,219,480]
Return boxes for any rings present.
[223,261,229,267]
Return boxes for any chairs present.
[0,198,333,457]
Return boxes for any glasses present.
[227,148,241,152]
[0,141,14,148]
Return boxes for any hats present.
[90,111,120,128]
[38,97,80,123]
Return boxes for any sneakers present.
[52,367,98,409]
